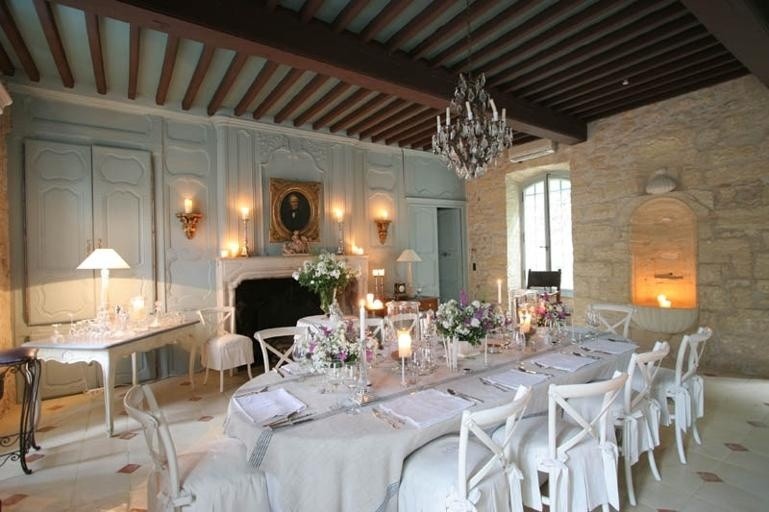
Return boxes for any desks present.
[369,294,437,317]
[294,315,359,352]
[22,313,200,439]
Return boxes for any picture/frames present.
[268,176,321,244]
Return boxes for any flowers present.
[436,299,501,347]
[292,251,362,309]
[303,320,377,367]
[529,292,569,329]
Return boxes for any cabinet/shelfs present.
[23,138,156,327]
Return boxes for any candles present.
[502,108,505,120]
[436,116,440,129]
[379,269,384,275]
[498,283,501,303]
[373,269,378,275]
[360,304,365,337]
[446,107,451,125]
[489,99,497,112]
[466,101,471,113]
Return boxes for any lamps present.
[373,210,391,245]
[431,0,514,182]
[396,249,423,299]
[175,199,203,239]
[240,206,251,255]
[75,248,130,311]
[335,210,345,254]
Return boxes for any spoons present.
[518,367,554,378]
[608,338,628,342]
[447,388,483,403]
[373,408,398,430]
[479,377,508,392]
[580,347,612,355]
[263,412,298,428]
[572,351,601,361]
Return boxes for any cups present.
[590,311,601,328]
[513,331,526,363]
[326,359,345,410]
[407,351,420,385]
[292,342,307,382]
[344,362,363,415]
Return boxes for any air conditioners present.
[508,138,555,163]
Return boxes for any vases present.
[536,327,549,338]
[319,287,335,315]
[458,338,483,358]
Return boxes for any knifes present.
[272,367,285,379]
[268,413,313,426]
[273,418,313,431]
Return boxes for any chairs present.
[492,369,628,512]
[561,341,671,507]
[349,318,384,346]
[398,383,533,512]
[386,312,420,338]
[254,326,309,373]
[589,305,633,339]
[198,306,254,392]
[527,268,561,306]
[631,326,711,465]
[123,384,269,512]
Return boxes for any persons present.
[283,194,309,231]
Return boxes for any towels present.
[235,389,308,426]
[282,362,309,374]
[483,368,550,391]
[581,338,639,355]
[537,352,598,373]
[561,324,589,334]
[380,388,476,427]
